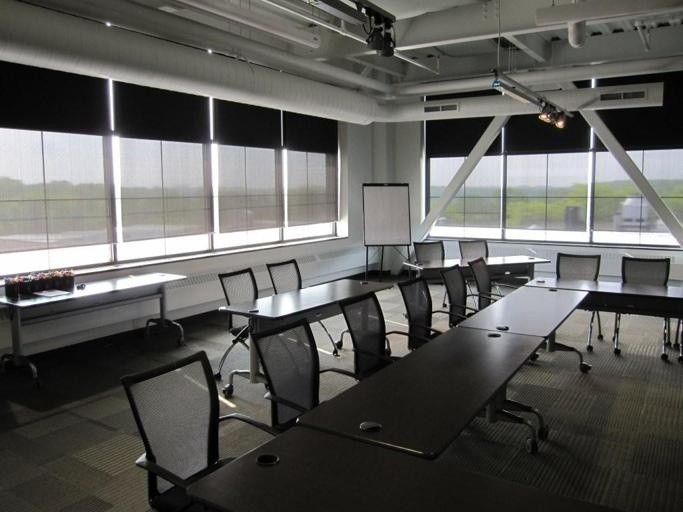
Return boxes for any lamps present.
[366,14,396,58]
[538,104,566,129]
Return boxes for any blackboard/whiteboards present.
[362,183,412,246]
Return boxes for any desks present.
[217,279,394,399]
[0,271,187,381]
[297,327,545,460]
[401,254,550,320]
[460,287,589,440]
[524,277,681,320]
[529,279,682,363]
[185,425,432,512]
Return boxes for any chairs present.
[338,293,435,380]
[265,259,341,363]
[458,240,501,302]
[252,320,365,427]
[413,241,448,307]
[215,268,301,382]
[610,256,671,360]
[399,279,469,351]
[121,349,280,511]
[555,252,602,350]
[466,256,520,309]
[441,263,497,329]
